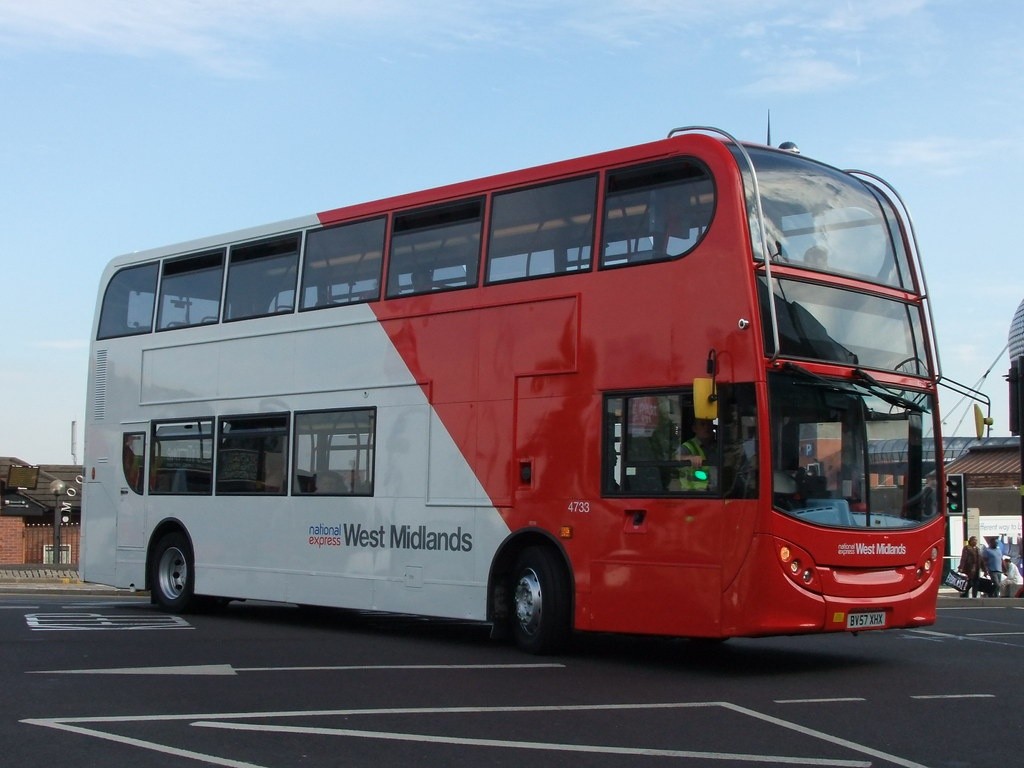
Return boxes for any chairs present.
[142,468,371,496]
[141,282,447,328]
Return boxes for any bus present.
[79,110,993,656]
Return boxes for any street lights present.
[50,480,66,564]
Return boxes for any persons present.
[623,416,744,498]
[999,555,1024,597]
[982,537,1007,598]
[957,536,988,599]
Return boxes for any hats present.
[1002,555,1010,560]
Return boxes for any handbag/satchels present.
[945,570,970,593]
[978,575,992,593]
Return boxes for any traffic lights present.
[921,487,936,516]
[946,474,965,516]
